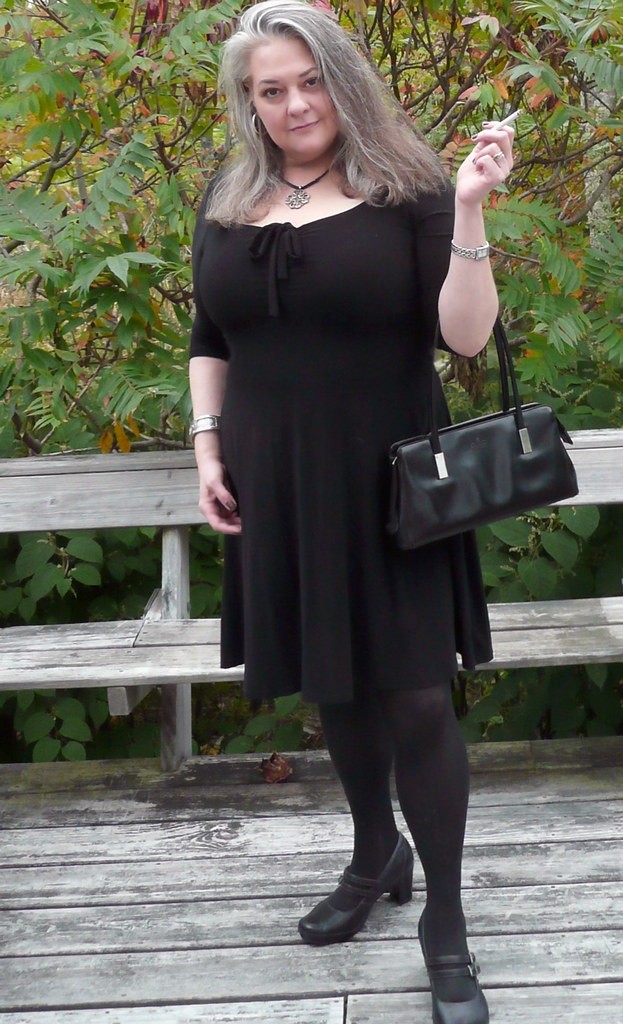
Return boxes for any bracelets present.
[188,415,221,447]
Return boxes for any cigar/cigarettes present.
[491,109,523,131]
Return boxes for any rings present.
[493,150,504,163]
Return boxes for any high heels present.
[298,831,415,944]
[417,909,490,1024]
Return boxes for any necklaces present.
[269,157,342,209]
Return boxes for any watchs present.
[450,241,490,260]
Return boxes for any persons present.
[188,0,517,1024]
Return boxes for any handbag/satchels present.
[388,313,579,550]
